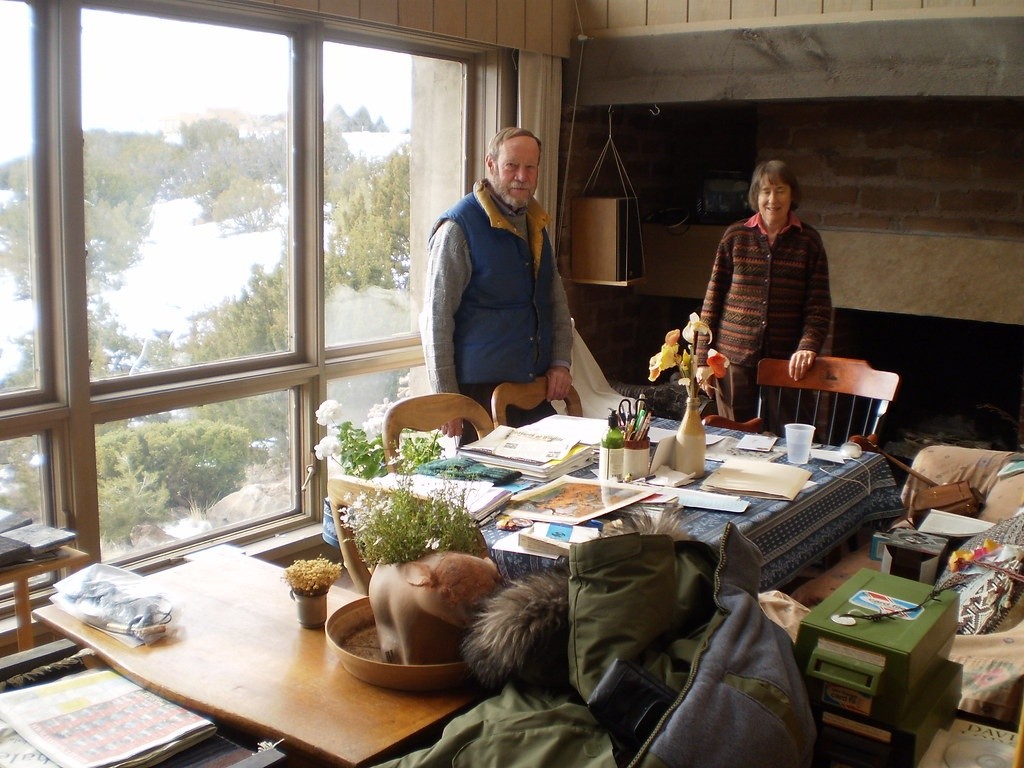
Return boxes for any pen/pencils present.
[633,474,656,482]
[625,409,652,440]
[638,394,645,414]
[812,444,823,448]
[478,506,506,528]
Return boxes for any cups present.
[784,423,815,465]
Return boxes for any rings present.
[802,361,806,365]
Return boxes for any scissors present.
[618,399,649,426]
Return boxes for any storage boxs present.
[795,567,963,767]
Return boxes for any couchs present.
[758,445,1024,724]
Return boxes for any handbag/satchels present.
[912,480,988,530]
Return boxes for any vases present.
[675,401,705,477]
[324,553,502,689]
[291,591,328,626]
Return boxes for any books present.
[457,424,595,484]
[378,471,512,528]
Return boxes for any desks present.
[34,550,470,768]
[0,545,90,651]
[421,417,904,593]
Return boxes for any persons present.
[424,128,574,445]
[698,157,832,436]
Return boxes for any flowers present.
[284,558,339,594]
[648,313,730,398]
[313,399,481,558]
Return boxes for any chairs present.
[702,356,902,450]
[383,393,494,473]
[491,377,583,430]
